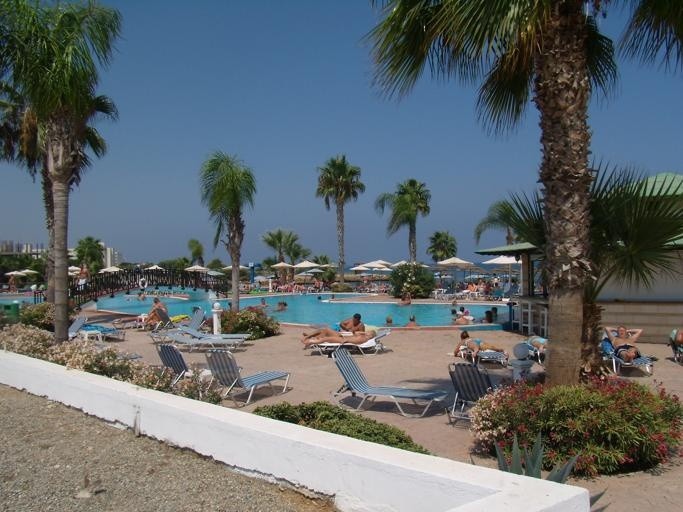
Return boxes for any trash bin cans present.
[1,303,19,325]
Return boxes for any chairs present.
[434,279,520,302]
[443,331,547,426]
[329,347,449,420]
[67,309,291,408]
[309,328,392,362]
[599,330,658,377]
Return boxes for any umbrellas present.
[268,254,524,298]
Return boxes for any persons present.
[603,325,644,363]
[4,264,543,346]
[526,332,547,354]
[669,328,683,345]
[453,329,504,358]
